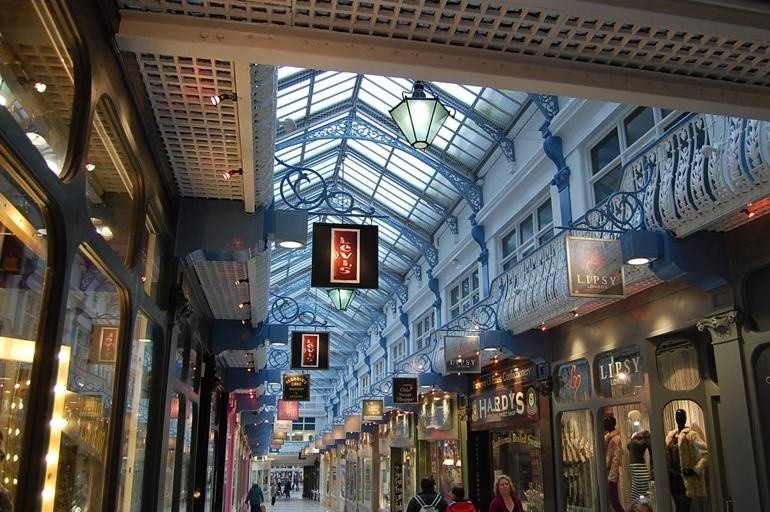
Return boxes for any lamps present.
[388,80,457,151]
[327,287,360,313]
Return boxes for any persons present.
[270,472,300,505]
[625,409,654,507]
[446,486,477,512]
[244,481,264,512]
[406,475,448,512]
[665,409,711,512]
[488,475,524,512]
[603,415,625,512]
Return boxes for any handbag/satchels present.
[260,506,266,512]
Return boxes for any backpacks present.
[414,495,442,512]
